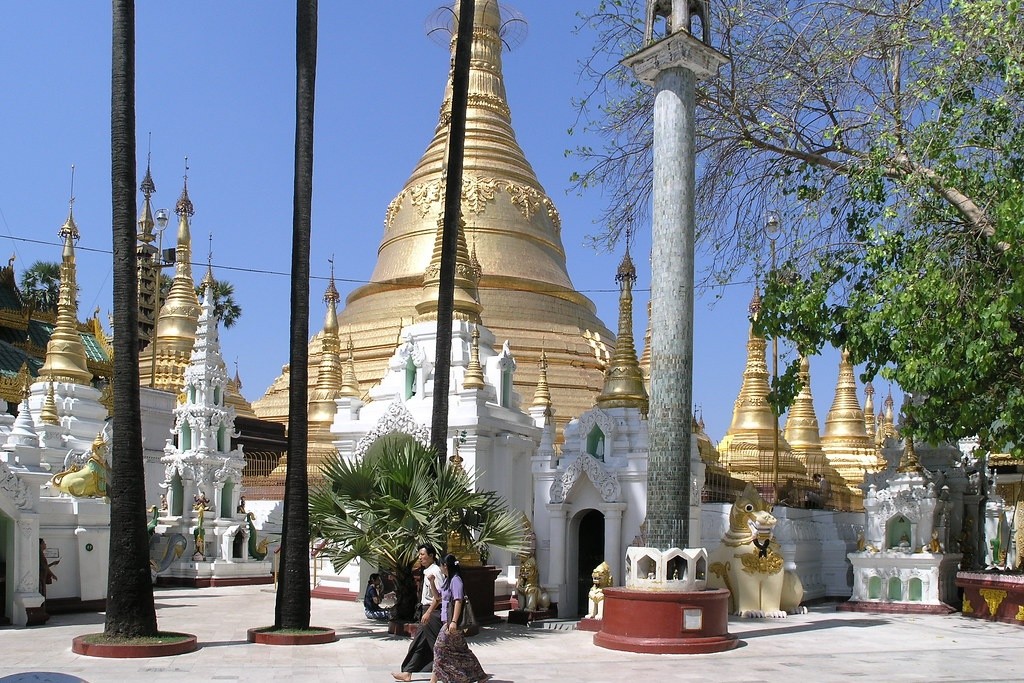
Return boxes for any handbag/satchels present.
[446,596,480,637]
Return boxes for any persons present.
[160,493,167,509]
[39,538,60,607]
[240,496,246,514]
[391,543,488,683]
[193,492,210,506]
[778,479,795,504]
[807,473,829,509]
[364,573,390,619]
